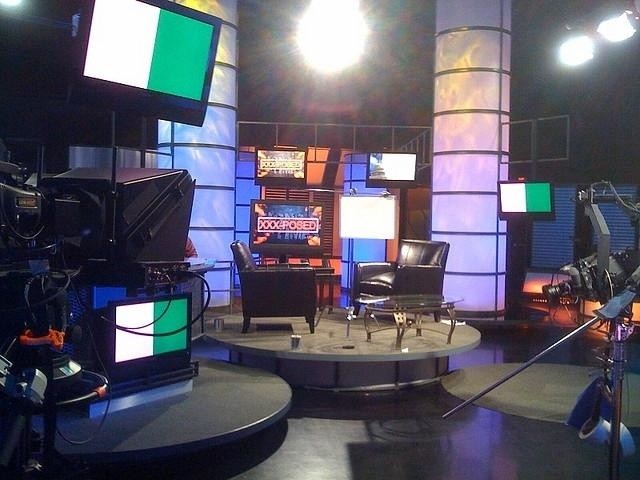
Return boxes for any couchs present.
[230,240,317,333]
[353,239,450,322]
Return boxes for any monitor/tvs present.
[106,292,192,373]
[254,147,308,188]
[366,152,417,188]
[248,199,326,257]
[67,0,222,127]
[497,181,555,221]
